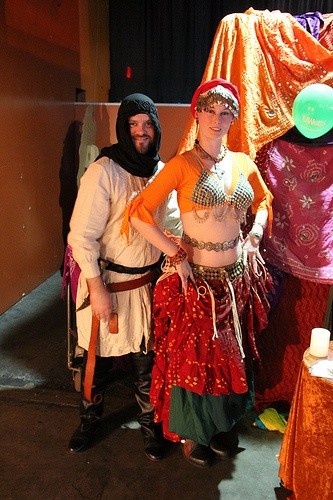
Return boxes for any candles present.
[309,328,330,357]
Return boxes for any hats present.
[191,78,239,121]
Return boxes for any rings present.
[252,258,256,260]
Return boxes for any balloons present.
[292,84,333,139]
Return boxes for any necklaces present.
[194,139,228,180]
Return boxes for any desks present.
[279,341,333,500]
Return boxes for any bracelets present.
[169,248,186,266]
[249,223,265,239]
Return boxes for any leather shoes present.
[182,436,210,468]
[141,423,165,461]
[69,419,101,452]
[209,432,232,460]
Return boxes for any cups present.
[309,327,331,357]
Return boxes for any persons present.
[128,81,274,468]
[60,93,183,461]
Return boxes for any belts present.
[98,254,164,274]
